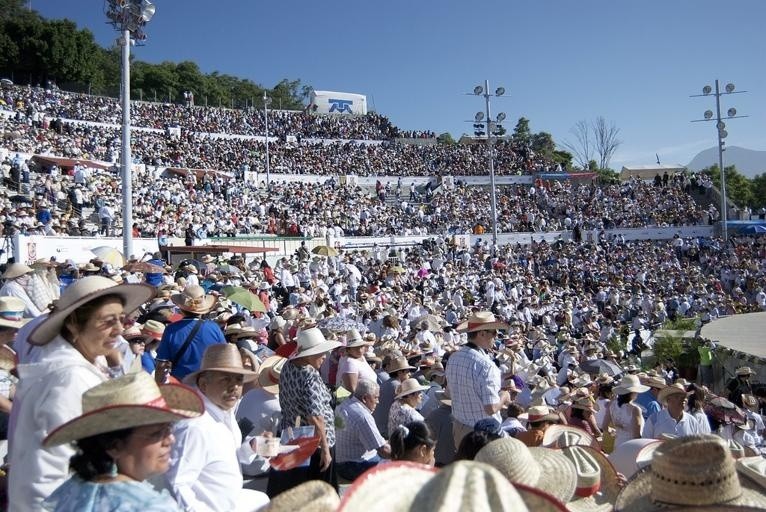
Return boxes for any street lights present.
[690,79,749,241]
[465,80,512,244]
[103,1,155,260]
[262,90,273,186]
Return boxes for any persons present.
[0,72,766,512]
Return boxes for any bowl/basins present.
[255,434,281,457]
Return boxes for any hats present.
[42,370,205,449]
[182,343,259,384]
[0,254,260,370]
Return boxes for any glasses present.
[143,420,178,441]
